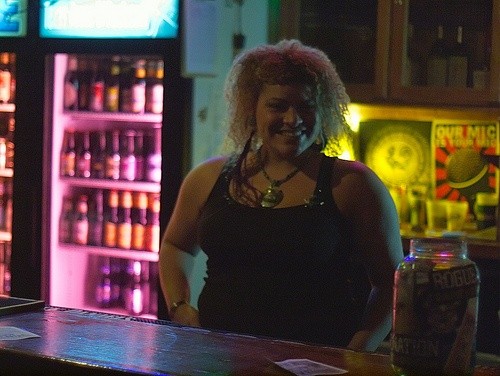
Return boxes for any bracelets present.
[169,301,187,322]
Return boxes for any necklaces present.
[257,144,311,208]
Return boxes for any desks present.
[0,303,500,376]
[398,220,498,250]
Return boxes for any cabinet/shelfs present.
[281,0,500,123]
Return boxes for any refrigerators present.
[0,0,34,299]
[34,0,189,319]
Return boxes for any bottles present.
[0,52,14,295]
[389,238,479,376]
[447,25,472,87]
[407,25,420,85]
[427,25,447,87]
[64,56,163,114]
[60,122,161,317]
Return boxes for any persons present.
[159,39,403,355]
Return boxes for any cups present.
[390,184,498,234]
